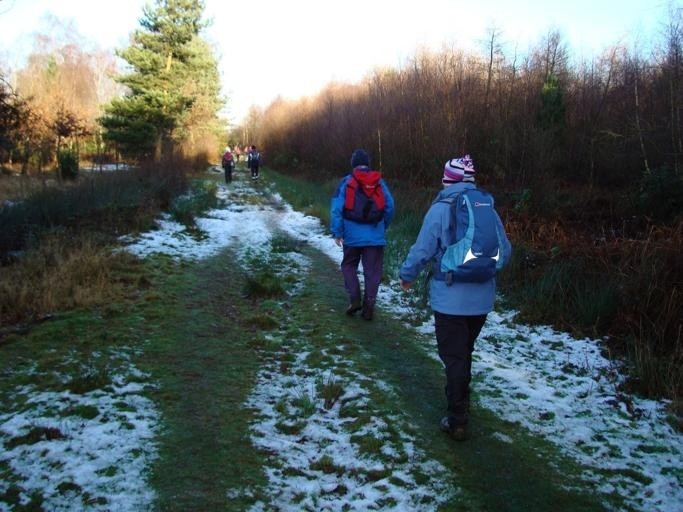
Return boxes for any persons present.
[327,145,396,321]
[396,153,513,443]
[220,142,262,184]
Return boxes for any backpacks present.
[224,152,232,162]
[432,188,511,283]
[343,170,385,223]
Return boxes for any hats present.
[442,155,475,185]
[350,150,369,167]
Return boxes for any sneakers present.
[441,417,468,441]
[347,301,372,320]
